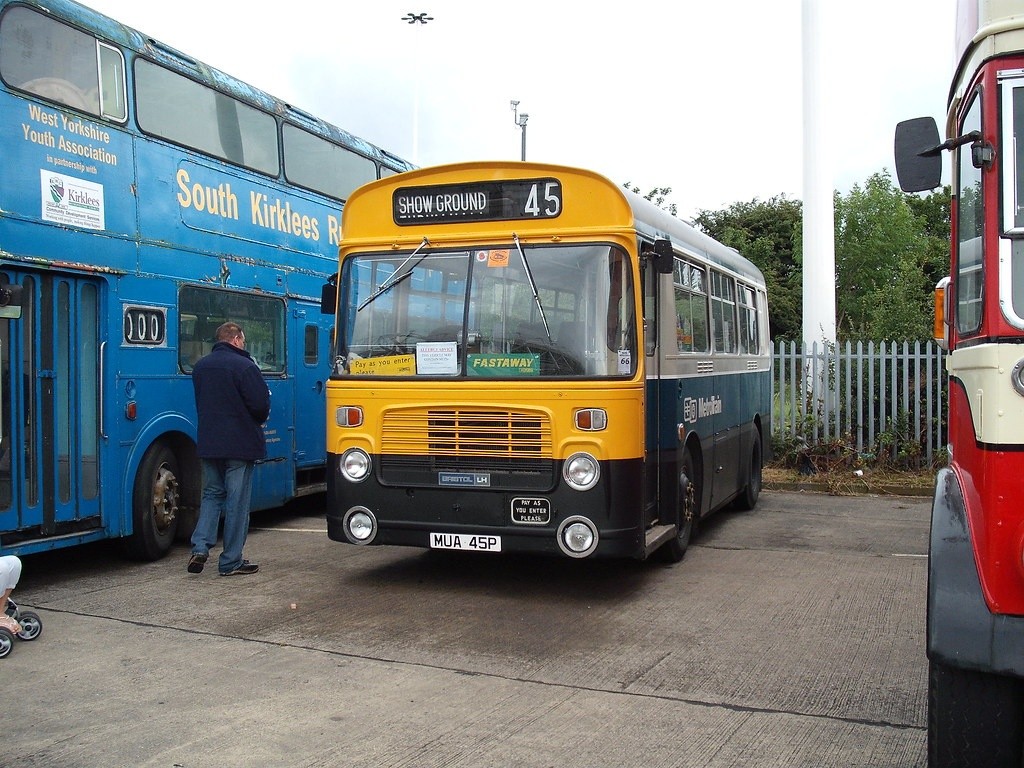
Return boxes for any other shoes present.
[0,615,22,634]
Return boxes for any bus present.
[0,0,583,564]
[895,0,1024,768]
[321,161,772,563]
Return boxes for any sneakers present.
[219,559,259,576]
[187,553,207,573]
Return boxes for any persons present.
[0,555,22,634]
[187,322,271,575]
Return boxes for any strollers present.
[0,595,43,659]
